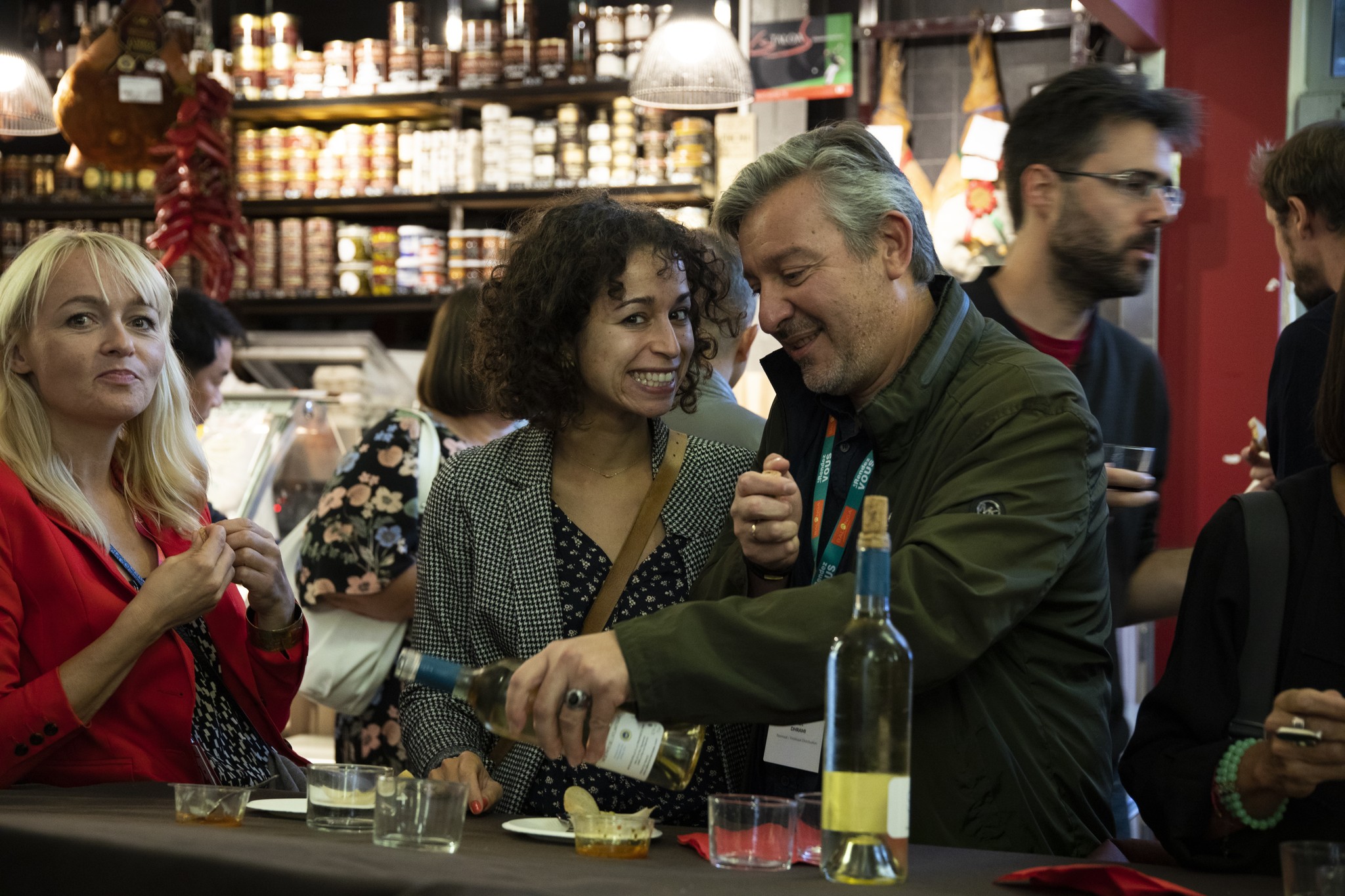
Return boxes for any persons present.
[1123,271,1345,869]
[280,285,531,773]
[0,225,315,783]
[504,119,1123,857]
[171,286,252,424]
[957,60,1277,845]
[1241,118,1344,492]
[394,185,758,826]
[655,228,769,454]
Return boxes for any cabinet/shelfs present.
[0,0,716,321]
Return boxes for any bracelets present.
[1215,736,1290,831]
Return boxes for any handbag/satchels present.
[278,409,443,716]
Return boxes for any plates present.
[308,793,374,810]
[247,797,309,816]
[500,814,663,843]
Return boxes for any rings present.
[565,688,592,709]
[752,520,759,542]
[1293,713,1306,729]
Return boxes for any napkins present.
[994,863,1201,895]
[677,819,823,866]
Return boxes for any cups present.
[304,762,393,835]
[570,811,655,860]
[796,789,822,863]
[373,774,470,855]
[1279,839,1345,895]
[168,782,252,827]
[707,796,794,872]
[1103,443,1156,493]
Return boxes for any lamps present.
[629,0,755,112]
[0,0,63,138]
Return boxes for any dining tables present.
[0,781,1345,895]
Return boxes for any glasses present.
[1049,168,1187,215]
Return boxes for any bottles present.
[0,0,717,300]
[824,493,915,886]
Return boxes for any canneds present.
[0,0,715,294]
